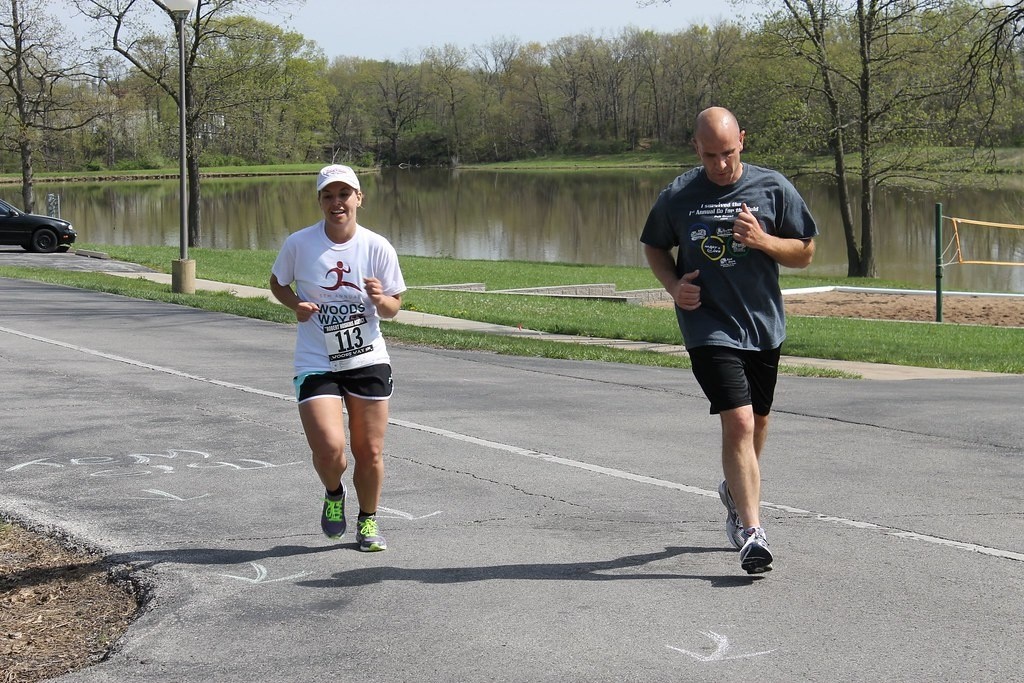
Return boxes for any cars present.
[0,198,77,253]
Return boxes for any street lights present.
[164,0,200,293]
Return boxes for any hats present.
[317,164,360,192]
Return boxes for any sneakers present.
[356,514,387,552]
[718,480,745,551]
[321,480,347,540]
[739,526,773,574]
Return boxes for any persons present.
[639,107,819,575]
[269,165,408,553]
